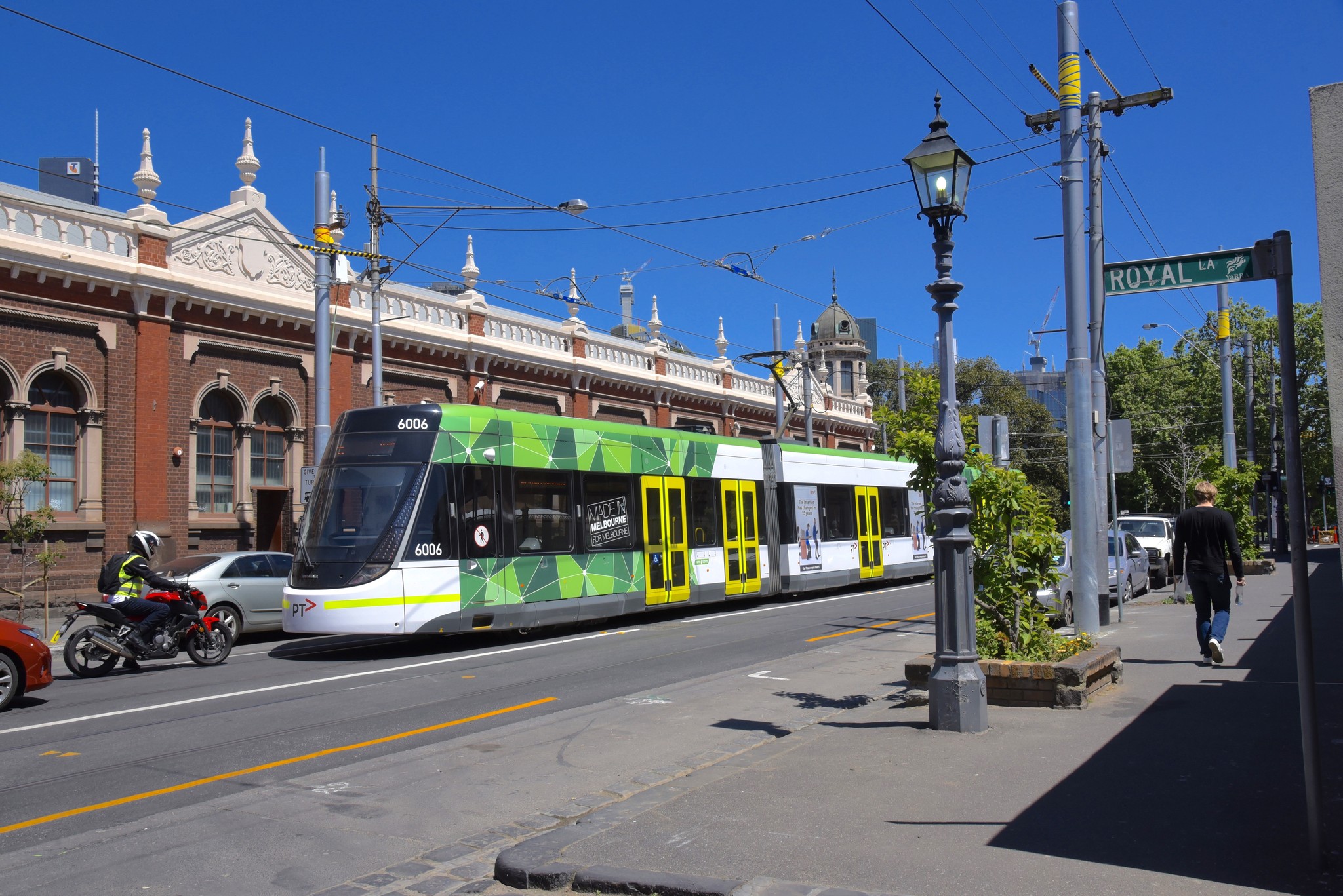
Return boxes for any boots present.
[122,654,142,670]
[125,624,150,650]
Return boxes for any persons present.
[805,523,812,561]
[797,526,801,557]
[921,516,927,550]
[1172,480,1245,666]
[911,523,913,547]
[107,531,191,651]
[813,518,821,560]
[916,521,921,551]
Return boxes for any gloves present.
[172,582,191,594]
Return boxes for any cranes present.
[1028,285,1060,358]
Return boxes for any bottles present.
[1235,583,1244,606]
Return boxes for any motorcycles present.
[57,567,232,678]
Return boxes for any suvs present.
[1105,512,1176,587]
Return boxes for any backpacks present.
[97,550,143,596]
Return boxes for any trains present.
[281,402,1011,641]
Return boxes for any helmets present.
[125,529,165,561]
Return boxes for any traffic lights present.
[1326,486,1336,495]
[1316,480,1324,495]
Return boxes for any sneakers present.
[1202,654,1214,663]
[1207,638,1225,666]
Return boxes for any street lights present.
[1272,433,1286,554]
[372,197,587,408]
[903,90,993,737]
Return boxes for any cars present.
[0,615,54,710]
[1059,527,1149,602]
[140,549,294,644]
[977,533,1085,627]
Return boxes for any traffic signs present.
[1104,246,1262,297]
